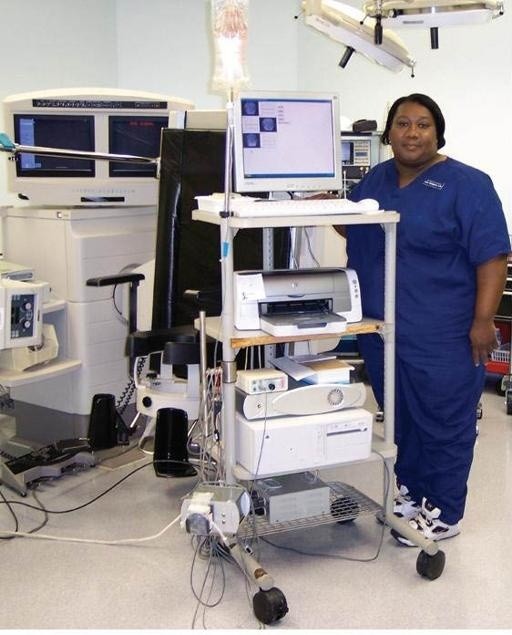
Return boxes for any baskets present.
[491,342,510,364]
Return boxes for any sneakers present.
[377,485,421,526]
[391,497,461,547]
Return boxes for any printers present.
[232,269,364,336]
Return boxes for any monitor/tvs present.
[12,111,98,180]
[106,114,170,179]
[235,91,345,200]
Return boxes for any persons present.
[302,94,512,547]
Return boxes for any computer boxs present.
[233,407,373,476]
